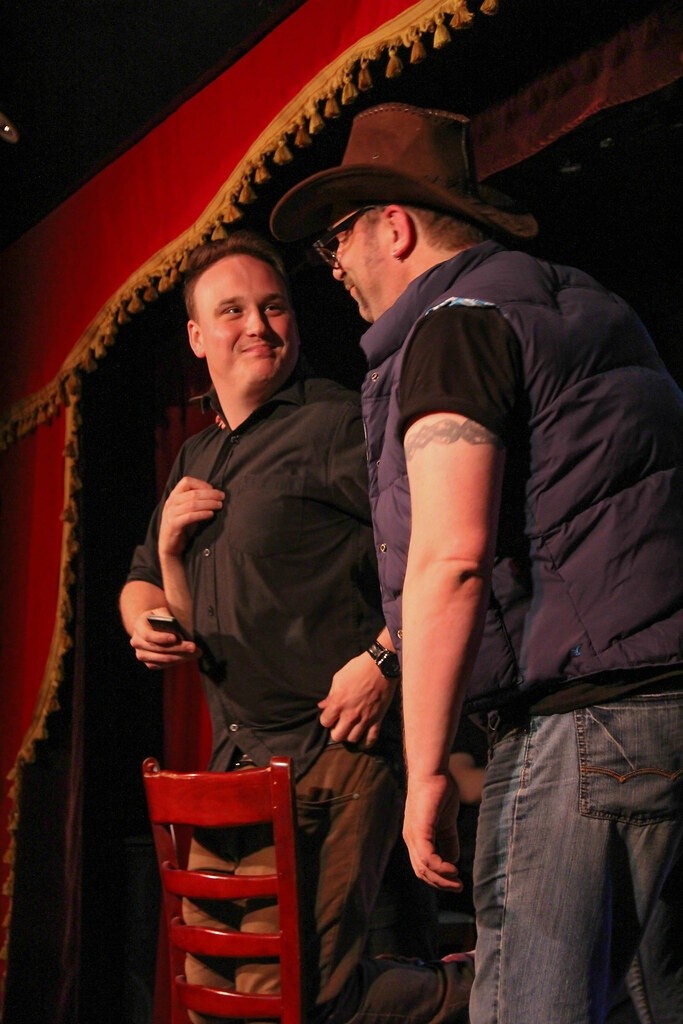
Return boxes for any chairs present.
[137,755,310,1024]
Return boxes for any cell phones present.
[147,614,182,646]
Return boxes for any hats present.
[268,105,539,250]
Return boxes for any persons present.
[271,103,683,1024]
[119,235,483,1024]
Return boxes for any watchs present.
[366,640,402,683]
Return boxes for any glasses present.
[314,205,377,269]
